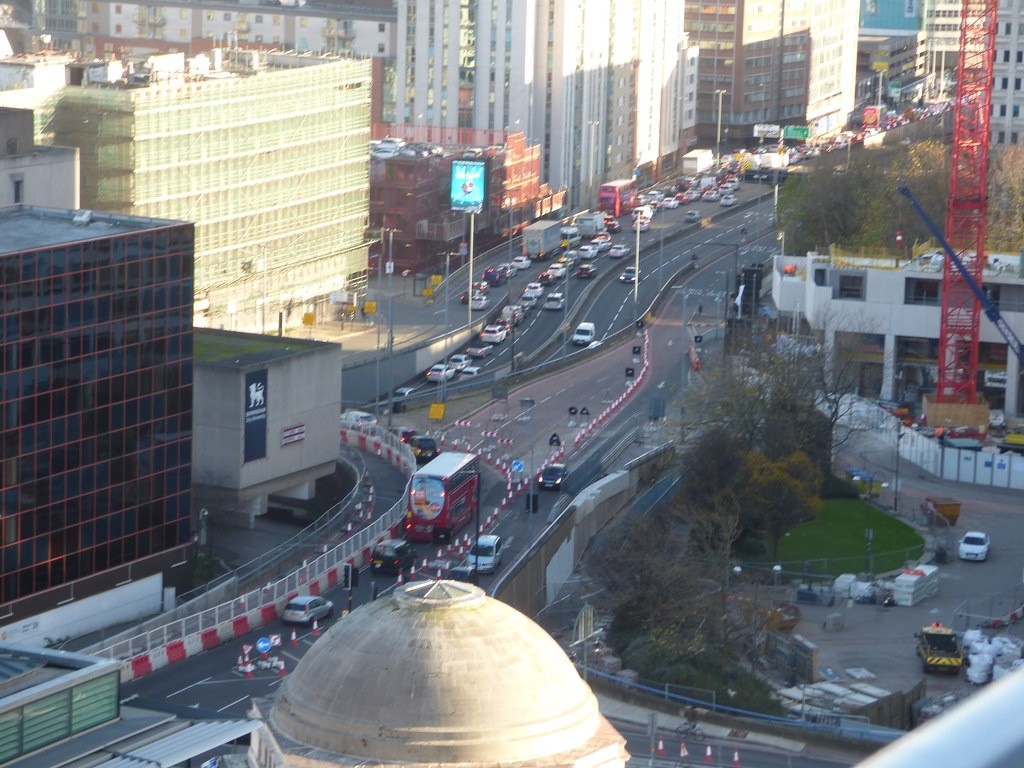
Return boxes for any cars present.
[424,212,630,382]
[283,596,333,624]
[467,535,502,575]
[371,137,502,158]
[371,539,417,573]
[340,411,440,462]
[537,464,568,491]
[598,145,779,230]
[959,532,990,561]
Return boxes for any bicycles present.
[675,719,705,741]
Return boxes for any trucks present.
[914,623,963,674]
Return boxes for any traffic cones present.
[237,620,319,676]
[706,746,712,763]
[733,752,739,768]
[657,738,665,756]
[347,485,374,534]
[396,329,649,588]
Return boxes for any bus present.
[405,452,481,545]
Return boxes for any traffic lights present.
[342,564,358,591]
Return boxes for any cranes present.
[895,0,1024,405]
